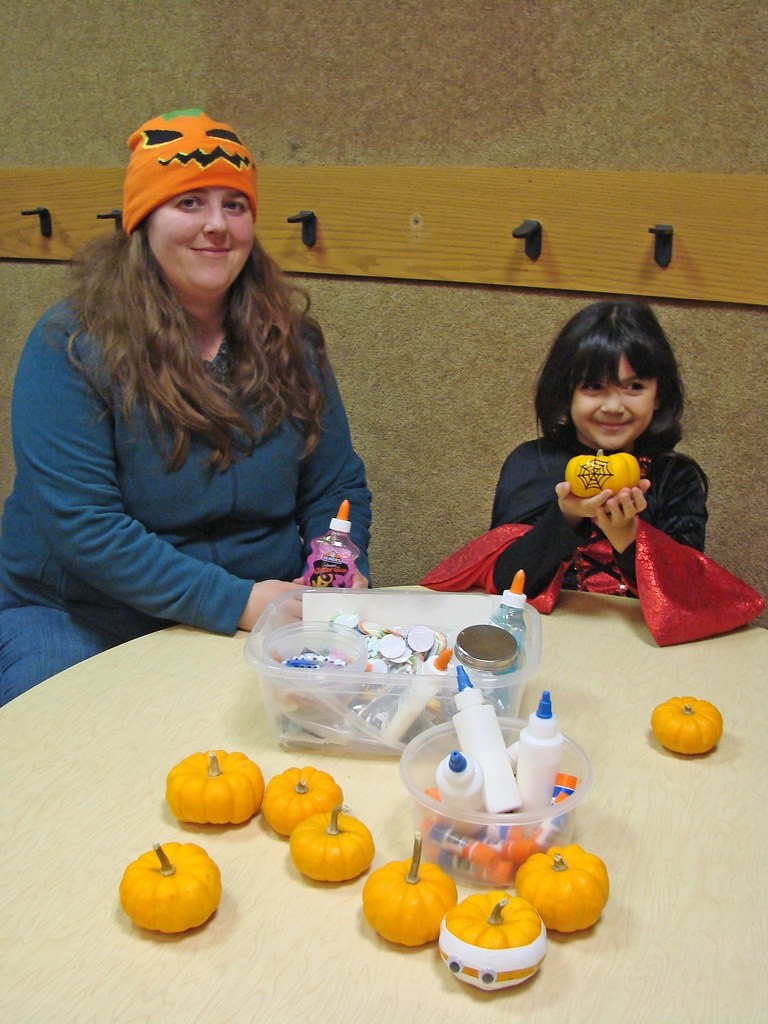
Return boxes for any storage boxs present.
[244,587,542,757]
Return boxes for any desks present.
[0,582,768,1024]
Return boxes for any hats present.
[122,108,258,238]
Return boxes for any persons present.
[490,297,709,602]
[0,105,373,710]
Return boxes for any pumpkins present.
[651,696,723,754]
[360,832,459,947]
[119,841,221,932]
[515,844,609,932]
[261,766,343,837]
[445,889,542,949]
[288,805,375,881]
[565,448,641,498]
[165,748,265,824]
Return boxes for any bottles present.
[304,500,359,589]
[487,570,527,707]
[440,625,518,722]
[513,691,563,835]
[436,750,486,834]
[453,665,522,812]
[381,649,453,742]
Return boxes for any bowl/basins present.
[400,716,591,886]
[263,620,367,715]
